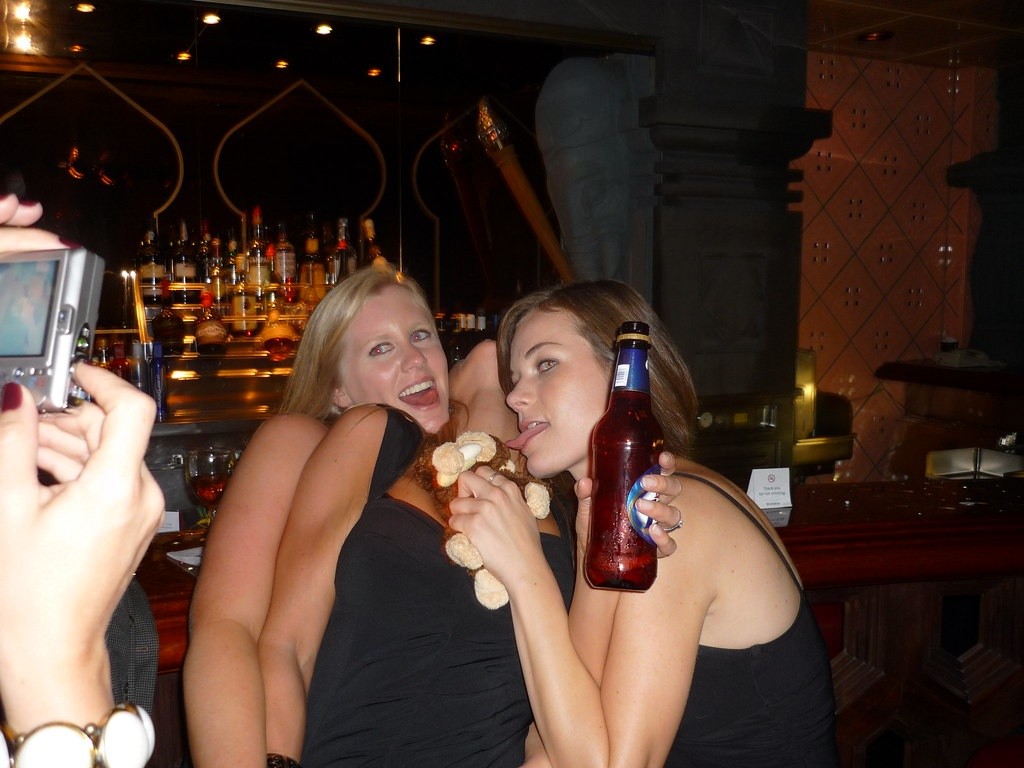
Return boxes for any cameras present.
[0,247,105,414]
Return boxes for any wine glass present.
[189,448,234,528]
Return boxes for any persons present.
[447,278,838,768]
[183,261,554,768]
[0,190,164,768]
[260,337,685,768]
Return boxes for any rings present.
[664,505,684,532]
[488,471,501,483]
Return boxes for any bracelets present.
[0,702,156,768]
[267,753,301,768]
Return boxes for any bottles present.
[93,204,381,421]
[433,312,488,370]
[583,321,664,593]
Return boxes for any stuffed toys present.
[412,430,554,611]
